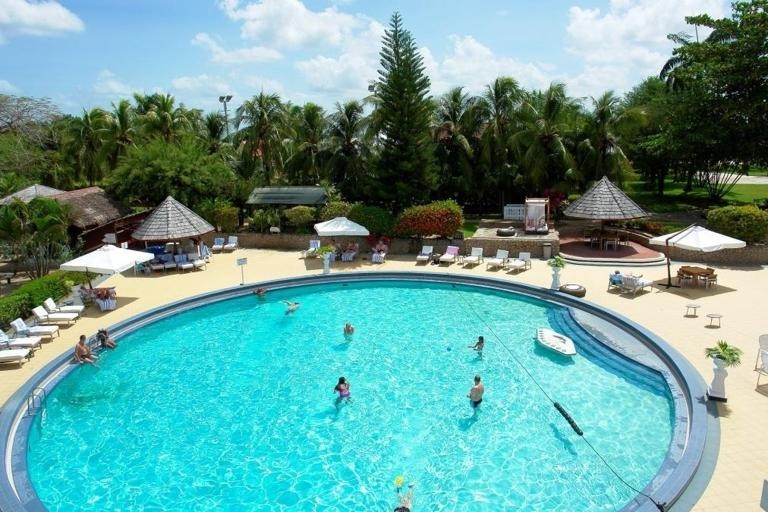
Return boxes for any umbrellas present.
[60,240,155,290]
[314,217,369,241]
[649,222,746,289]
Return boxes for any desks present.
[708,314,722,327]
[686,305,700,316]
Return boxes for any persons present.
[343,323,354,342]
[468,336,484,359]
[253,288,266,303]
[96,329,118,349]
[394,475,416,512]
[466,374,484,417]
[285,299,300,316]
[96,290,110,311]
[80,286,116,294]
[342,241,357,253]
[610,270,644,285]
[74,335,100,369]
[333,377,350,415]
[372,240,385,255]
[331,240,341,255]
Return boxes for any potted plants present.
[705,341,744,402]
[548,256,567,291]
[319,245,335,274]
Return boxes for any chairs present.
[416,246,532,274]
[678,265,717,290]
[133,236,239,274]
[590,228,621,251]
[753,335,768,390]
[608,274,654,298]
[0,286,116,368]
[306,239,389,262]
